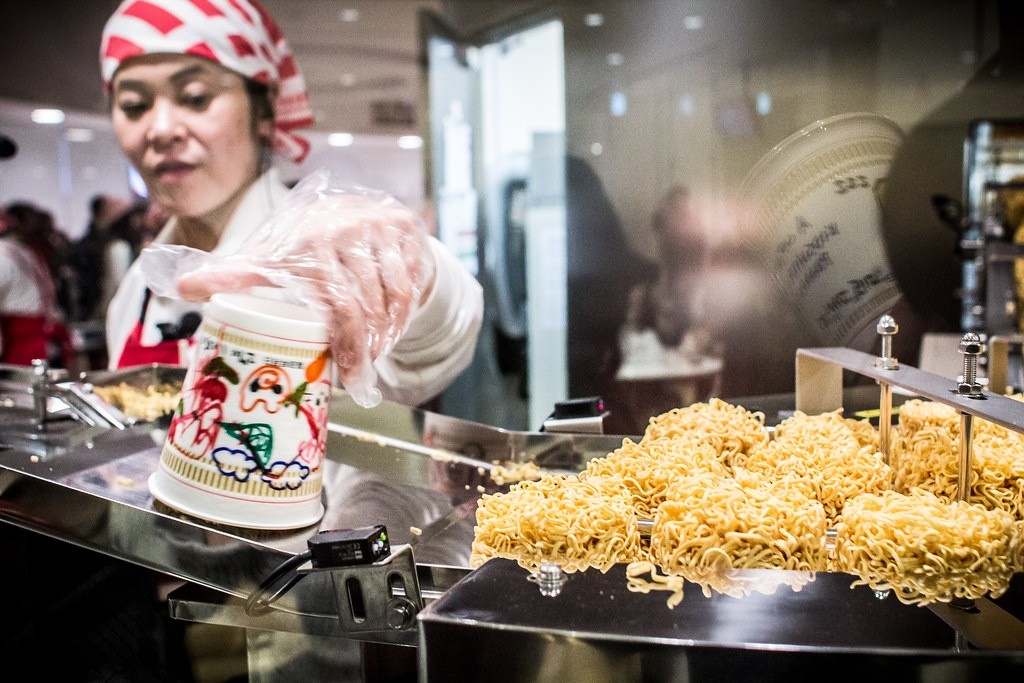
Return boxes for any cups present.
[734,111,925,359]
[146,292,335,528]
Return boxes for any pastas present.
[88,377,185,424]
[471,394,1024,609]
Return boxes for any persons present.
[624,185,776,361]
[0,134,172,386]
[95,0,485,409]
